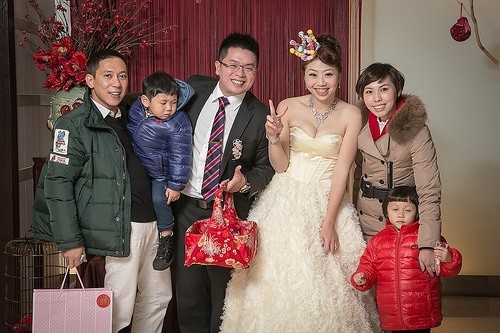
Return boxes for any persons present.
[352,62,442,276]
[30,48,173,333]
[218,34,381,333]
[126,70,193,271]
[167,33,276,333]
[351,186,463,333]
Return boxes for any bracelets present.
[266,133,280,144]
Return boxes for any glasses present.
[218,61,257,74]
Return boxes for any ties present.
[201,97,230,203]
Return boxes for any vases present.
[48,86,87,130]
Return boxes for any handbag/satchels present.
[32,266,113,333]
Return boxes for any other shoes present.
[153,232,176,270]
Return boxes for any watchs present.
[240,175,252,193]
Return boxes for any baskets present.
[184,183,259,269]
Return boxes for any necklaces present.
[307,93,338,126]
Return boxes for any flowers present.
[18,1,178,89]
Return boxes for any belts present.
[181,195,215,210]
